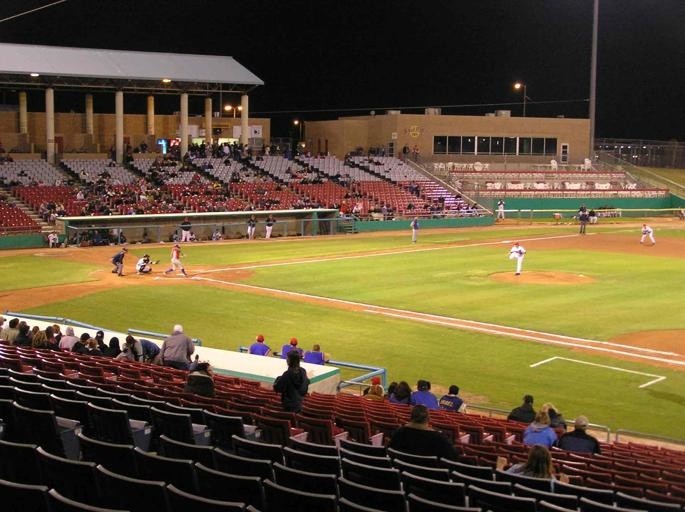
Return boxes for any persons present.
[1,315,216,399]
[136,254,160,274]
[495,394,602,482]
[410,217,420,243]
[48,232,58,248]
[143,228,150,243]
[72,230,126,244]
[172,217,195,242]
[496,198,506,221]
[508,242,526,277]
[2,137,479,225]
[163,244,190,277]
[247,215,258,239]
[265,214,276,239]
[361,375,467,461]
[213,229,222,240]
[640,224,655,245]
[111,248,129,277]
[578,206,598,234]
[247,333,327,414]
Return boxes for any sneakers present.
[111,270,190,278]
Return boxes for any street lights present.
[223,103,244,119]
[513,81,528,117]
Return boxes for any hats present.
[122,244,183,257]
[290,338,298,345]
[172,325,184,333]
[370,376,381,383]
[574,415,589,430]
[80,329,105,341]
[256,334,264,342]
